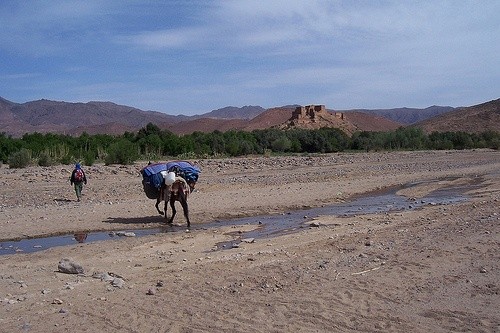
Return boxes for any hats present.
[76,163,81,167]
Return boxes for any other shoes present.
[78,197,81,202]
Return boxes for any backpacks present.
[74,169,84,181]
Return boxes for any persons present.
[70,163,88,202]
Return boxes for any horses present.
[147,161,190,228]
